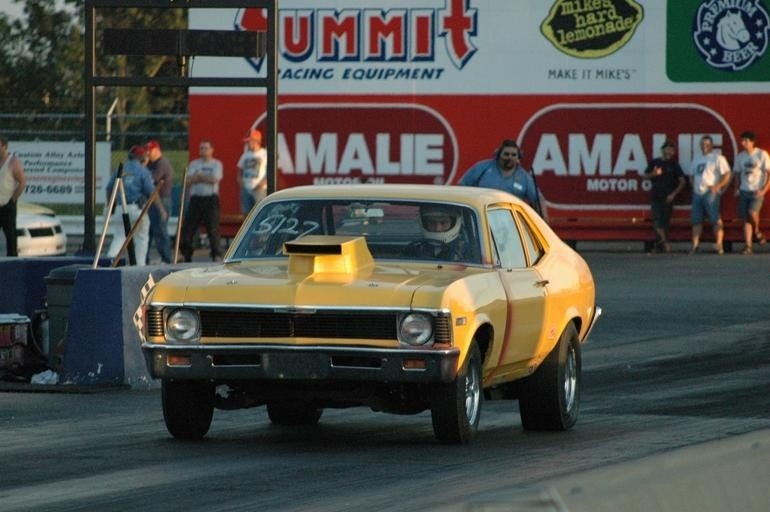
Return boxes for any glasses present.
[504,152,516,156]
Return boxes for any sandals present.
[740,230,766,255]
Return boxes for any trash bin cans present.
[43,264,98,372]
[0,313,31,370]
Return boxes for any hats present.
[129,140,160,155]
[242,129,262,142]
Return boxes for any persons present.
[642,142,686,255]
[732,131,770,256]
[685,136,731,258]
[456,139,549,225]
[235,130,268,222]
[0,135,25,257]
[396,203,481,264]
[104,144,168,267]
[176,139,225,263]
[144,141,172,266]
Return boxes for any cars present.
[16,202,67,256]
[141,183,602,445]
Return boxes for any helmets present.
[419,204,464,246]
